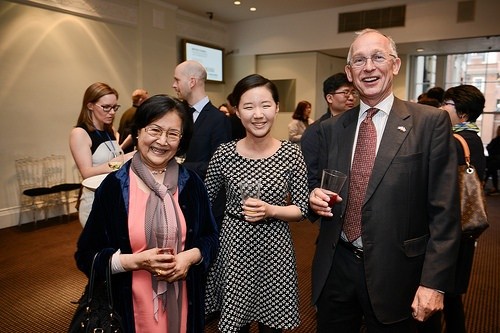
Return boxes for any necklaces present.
[151,168,167,174]
[96,129,117,157]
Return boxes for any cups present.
[240,179,261,214]
[154,226,178,264]
[320,168,348,211]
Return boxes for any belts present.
[224,210,278,225]
[340,240,364,261]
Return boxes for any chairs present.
[14,155,63,229]
[40,154,83,224]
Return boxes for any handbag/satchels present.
[69,252,124,332]
[452,132,489,234]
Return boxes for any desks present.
[81,172,111,195]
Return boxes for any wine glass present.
[108,150,124,171]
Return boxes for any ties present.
[342,107,380,243]
[187,107,196,124]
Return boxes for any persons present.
[218,103,232,117]
[430,82,488,333]
[302,27,460,333]
[288,99,314,151]
[227,92,247,142]
[116,88,151,155]
[417,86,447,106]
[71,82,125,229]
[486,124,500,196]
[173,61,233,328]
[76,93,222,332]
[301,73,360,159]
[204,73,310,333]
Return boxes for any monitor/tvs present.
[182,38,225,83]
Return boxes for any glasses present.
[93,102,121,112]
[347,51,396,67]
[437,101,455,108]
[330,90,357,97]
